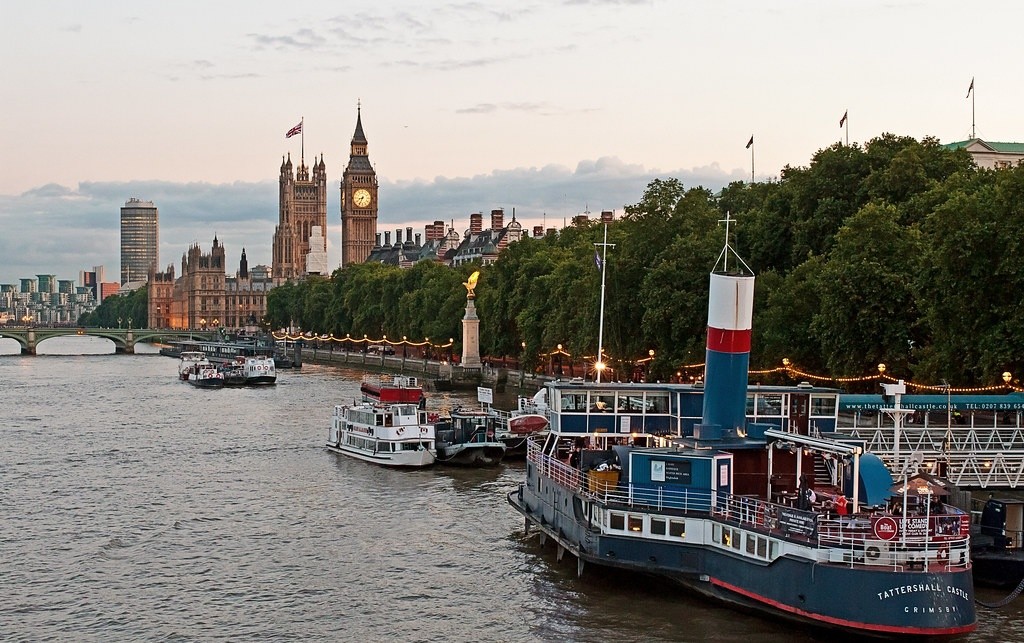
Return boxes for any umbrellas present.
[888,471,959,511]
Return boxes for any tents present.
[838,394,1024,425]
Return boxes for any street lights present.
[449,337,454,364]
[346,334,350,361]
[329,333,333,361]
[363,335,367,357]
[648,349,654,384]
[403,335,407,359]
[521,342,526,369]
[314,333,317,354]
[1001,372,1013,427]
[879,363,886,396]
[782,358,790,386]
[425,337,429,363]
[557,343,563,383]
[383,335,387,358]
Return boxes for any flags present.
[840,112,847,128]
[745,137,753,149]
[966,79,973,98]
[285,122,301,138]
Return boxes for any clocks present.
[354,189,371,207]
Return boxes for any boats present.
[361,372,423,408]
[178,351,277,388]
[507,415,548,432]
[326,397,506,469]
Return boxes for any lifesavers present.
[427,413,439,422]
[211,370,214,373]
[937,543,949,564]
[264,365,270,370]
[216,374,221,378]
[208,373,213,378]
[257,366,261,370]
[203,369,208,373]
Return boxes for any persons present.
[428,413,440,423]
[570,444,603,469]
[943,521,959,535]
[890,496,947,517]
[826,490,847,515]
[805,488,816,511]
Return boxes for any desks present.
[588,470,619,494]
[772,489,926,517]
